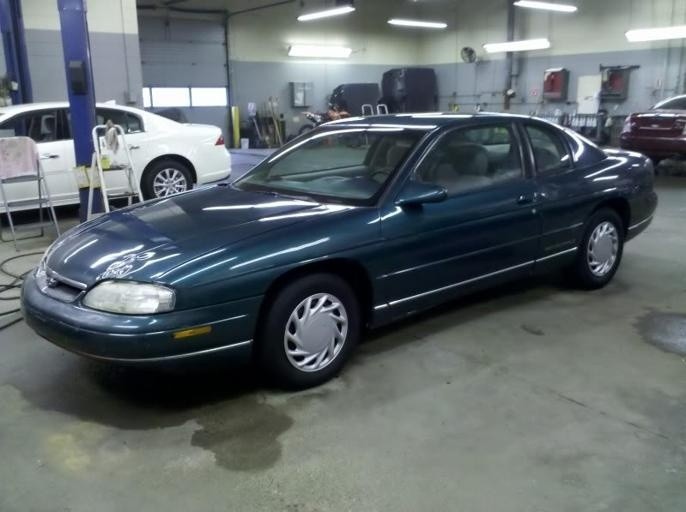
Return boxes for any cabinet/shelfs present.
[597,63,630,101]
[543,67,569,102]
[289,80,315,108]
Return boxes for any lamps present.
[286,44,353,59]
[512,0,578,14]
[482,37,552,54]
[386,18,448,30]
[296,1,356,24]
[624,25,686,43]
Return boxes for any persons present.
[328,109,337,120]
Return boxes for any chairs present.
[373,143,548,193]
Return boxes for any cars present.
[620,93,686,166]
[0,98,231,215]
[16,109,663,394]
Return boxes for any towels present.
[0,136,39,183]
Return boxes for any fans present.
[460,46,481,66]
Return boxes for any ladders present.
[86,124,145,221]
[0,136,61,250]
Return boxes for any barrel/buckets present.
[241,137,249,150]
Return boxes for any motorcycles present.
[298,105,361,150]
